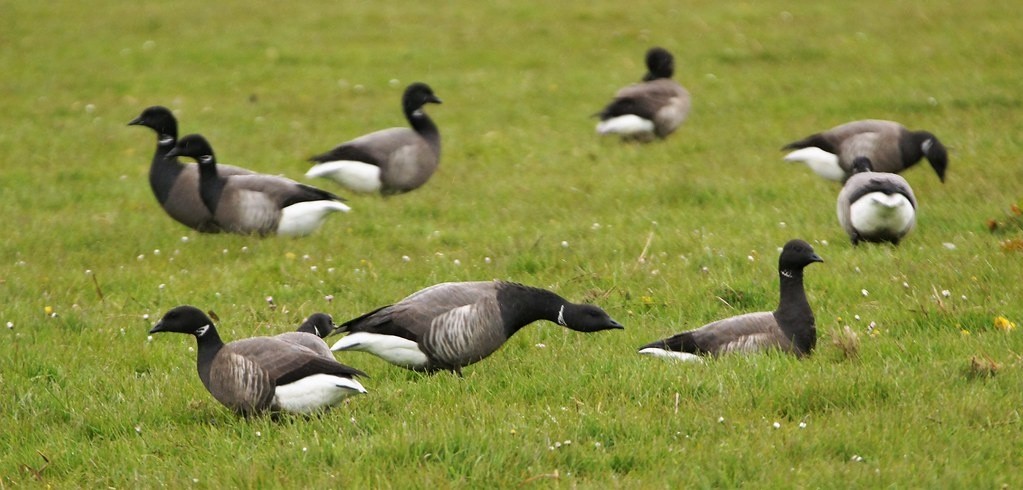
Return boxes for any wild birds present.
[778,118,949,185]
[304,81,444,197]
[588,46,691,144]
[329,280,625,377]
[636,238,825,366]
[835,156,917,245]
[272,313,340,363]
[126,106,258,235]
[165,133,351,240]
[149,306,372,423]
